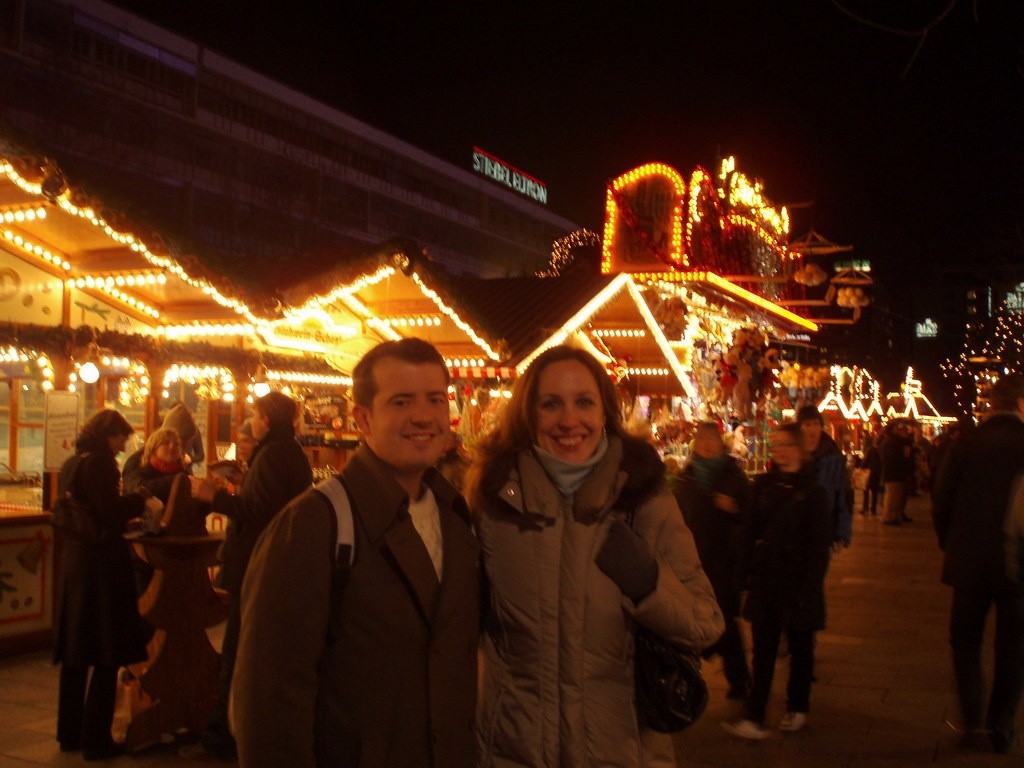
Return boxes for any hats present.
[161,403,196,438]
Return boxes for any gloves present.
[594,519,658,601]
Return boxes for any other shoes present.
[859,510,865,515]
[779,712,807,731]
[83,741,125,759]
[902,515,913,524]
[727,674,751,699]
[944,705,976,733]
[988,727,1012,753]
[727,721,763,739]
[60,742,81,751]
[871,509,876,515]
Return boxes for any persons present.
[855,417,932,528]
[50,391,314,761]
[931,372,1024,754]
[228,337,725,768]
[671,402,855,739]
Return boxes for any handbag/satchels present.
[629,507,710,735]
[851,466,871,491]
